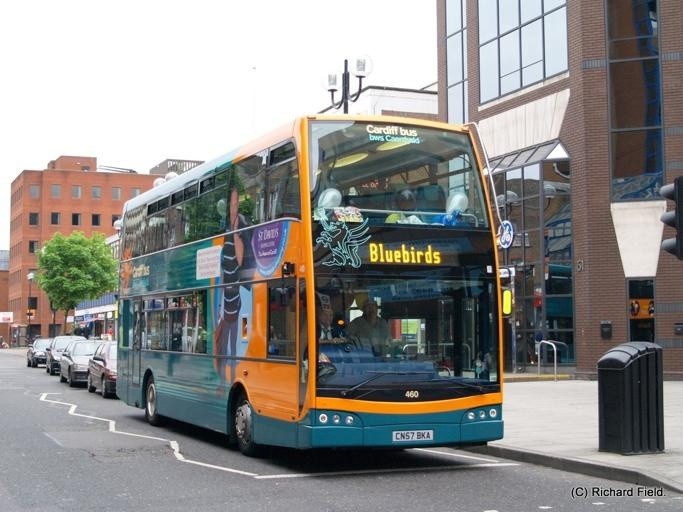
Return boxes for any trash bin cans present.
[596,341,664,456]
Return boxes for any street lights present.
[542,182,558,215]
[492,188,520,266]
[25,272,35,341]
[322,47,368,115]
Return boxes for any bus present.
[109,110,514,461]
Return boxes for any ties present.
[323,329,329,340]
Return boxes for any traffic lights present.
[656,172,682,263]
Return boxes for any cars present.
[123,317,209,354]
[22,334,118,401]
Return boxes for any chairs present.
[344,184,447,224]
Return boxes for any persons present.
[82,323,90,339]
[316,304,348,342]
[342,298,392,345]
[212,187,251,401]
